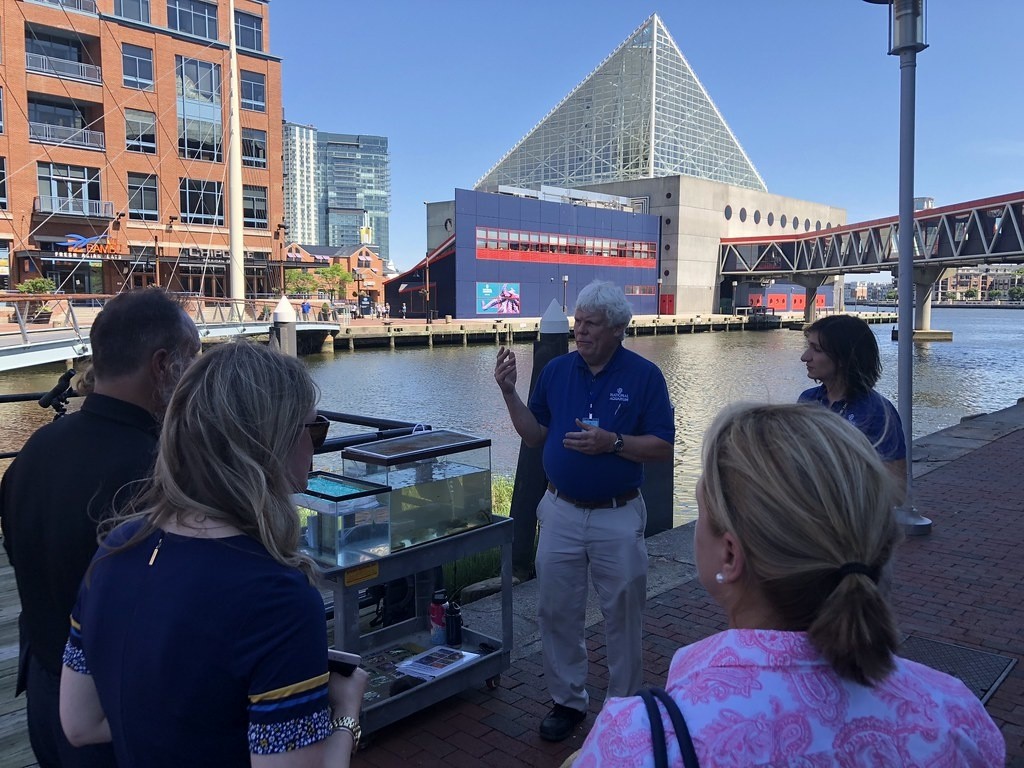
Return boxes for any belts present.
[546,481,639,510]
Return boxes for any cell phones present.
[327,649,362,679]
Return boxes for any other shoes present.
[539,700,587,739]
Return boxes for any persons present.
[386,303,390,314]
[797,315,907,506]
[301,302,310,321]
[402,303,406,316]
[561,402,1005,768]
[494,278,675,738]
[59,344,368,768]
[0,288,202,768]
[352,304,357,319]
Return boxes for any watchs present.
[614,432,623,457]
[331,716,361,755]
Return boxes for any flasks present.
[430,588,449,644]
[445,601,462,648]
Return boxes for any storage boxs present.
[340,428,492,554]
[293,470,392,568]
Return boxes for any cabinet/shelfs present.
[314,513,515,752]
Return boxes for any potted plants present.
[260,304,272,321]
[14,277,57,324]
[322,303,331,321]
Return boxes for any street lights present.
[876,286,881,313]
[732,281,738,317]
[863,0,934,537]
[561,274,569,315]
[854,288,859,311]
[656,279,662,319]
[893,287,898,313]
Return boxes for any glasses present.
[294,414,330,449]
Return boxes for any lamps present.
[274,224,286,239]
[166,216,178,233]
[112,211,126,230]
